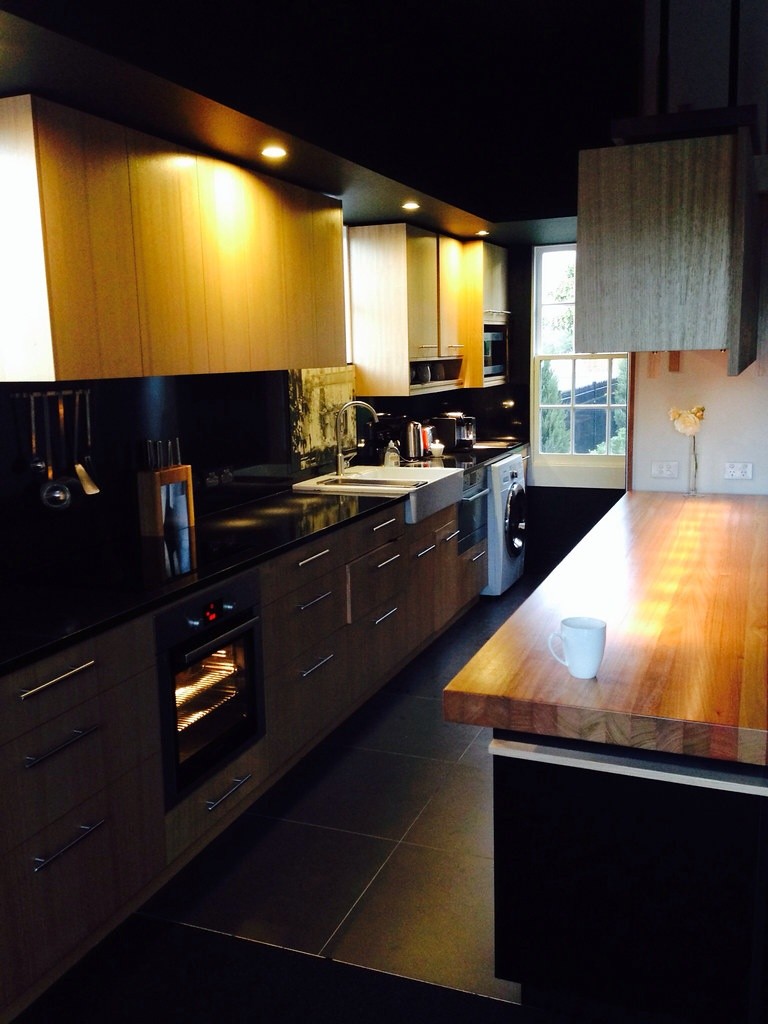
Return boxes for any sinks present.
[352,466,465,480]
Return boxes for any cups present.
[431,362,448,381]
[431,439,445,457]
[547,617,606,679]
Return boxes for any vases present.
[689,455,698,494]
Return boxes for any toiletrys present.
[384,440,400,467]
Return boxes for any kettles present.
[403,420,423,459]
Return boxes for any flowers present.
[668,406,706,450]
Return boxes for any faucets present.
[335,400,379,476]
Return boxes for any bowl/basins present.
[411,362,431,382]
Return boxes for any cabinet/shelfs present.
[0,95,509,397]
[575,124,768,377]
[442,491,767,769]
[0,473,487,1023]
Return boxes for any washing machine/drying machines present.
[480,453,529,596]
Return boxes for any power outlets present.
[723,463,752,480]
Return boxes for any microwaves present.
[484,332,505,375]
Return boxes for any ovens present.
[153,568,269,816]
[457,466,491,556]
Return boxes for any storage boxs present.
[137,465,197,582]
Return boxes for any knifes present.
[145,437,181,470]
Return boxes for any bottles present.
[456,417,477,441]
[385,440,400,467]
[422,419,434,456]
